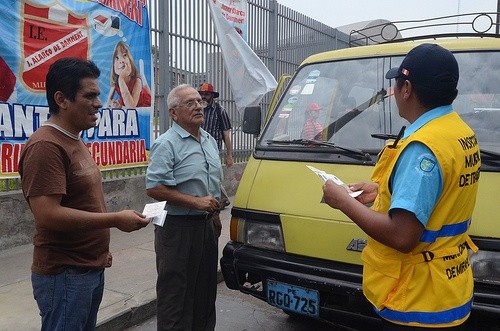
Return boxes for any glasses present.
[173,100,209,109]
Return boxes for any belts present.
[169,213,212,221]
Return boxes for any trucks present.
[219,36,500,331]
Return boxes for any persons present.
[299,103,324,143]
[146,83,223,331]
[106,42,151,109]
[196,83,233,209]
[18,57,151,331]
[323,43,481,331]
[333,97,363,150]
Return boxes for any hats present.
[385,42,459,97]
[197,82,219,98]
